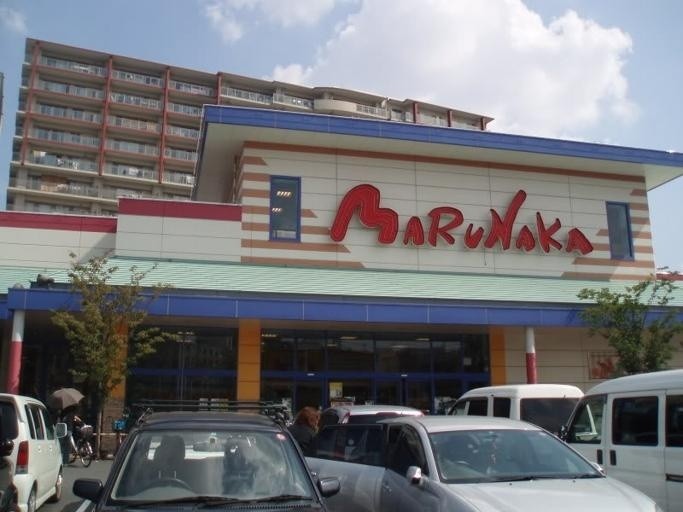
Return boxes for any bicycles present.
[65,425,94,468]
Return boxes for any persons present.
[59,407,86,467]
[287,407,320,456]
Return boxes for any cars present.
[311,404,428,434]
[73,397,341,512]
[307,415,662,510]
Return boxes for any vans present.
[0,391,66,512]
[446,383,598,439]
[553,371,682,510]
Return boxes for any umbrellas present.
[47,388,84,410]
